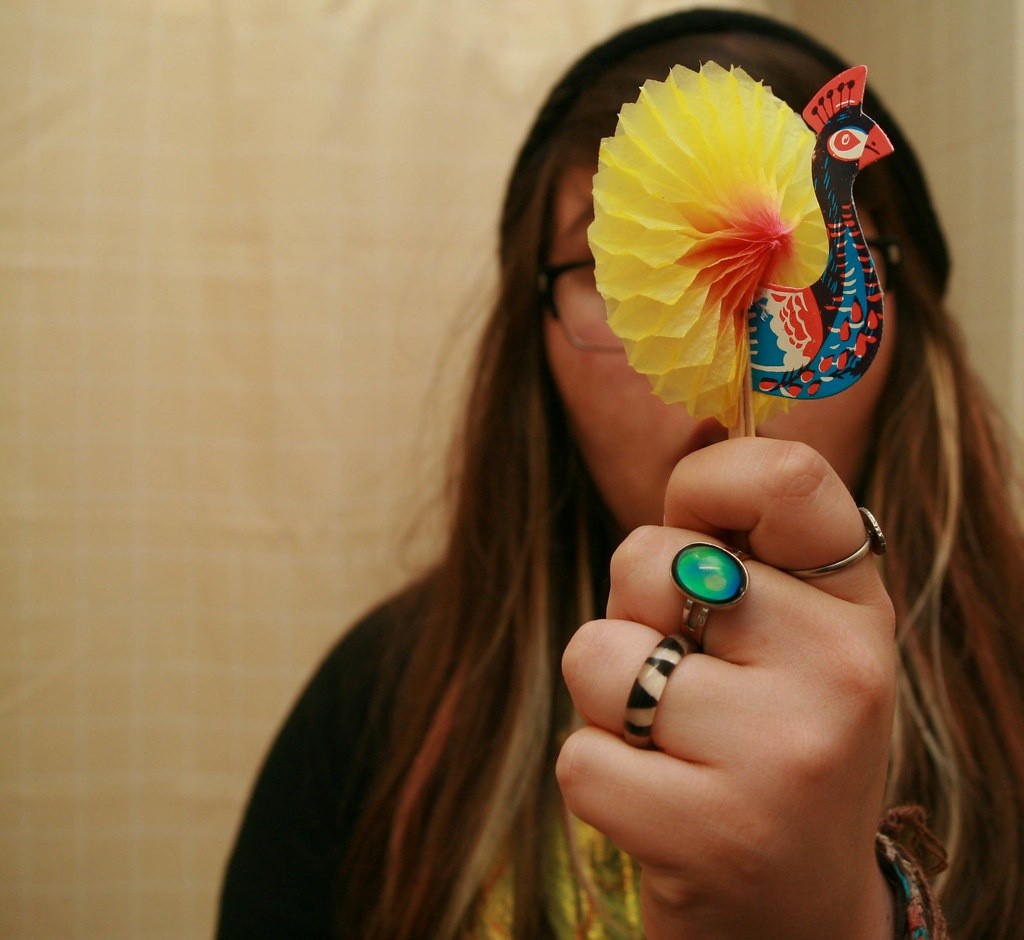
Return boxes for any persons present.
[210,5,1024,940]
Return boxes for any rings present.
[671,541,754,651]
[790,507,886,579]
[624,634,699,748]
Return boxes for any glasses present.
[535,237,899,351]
